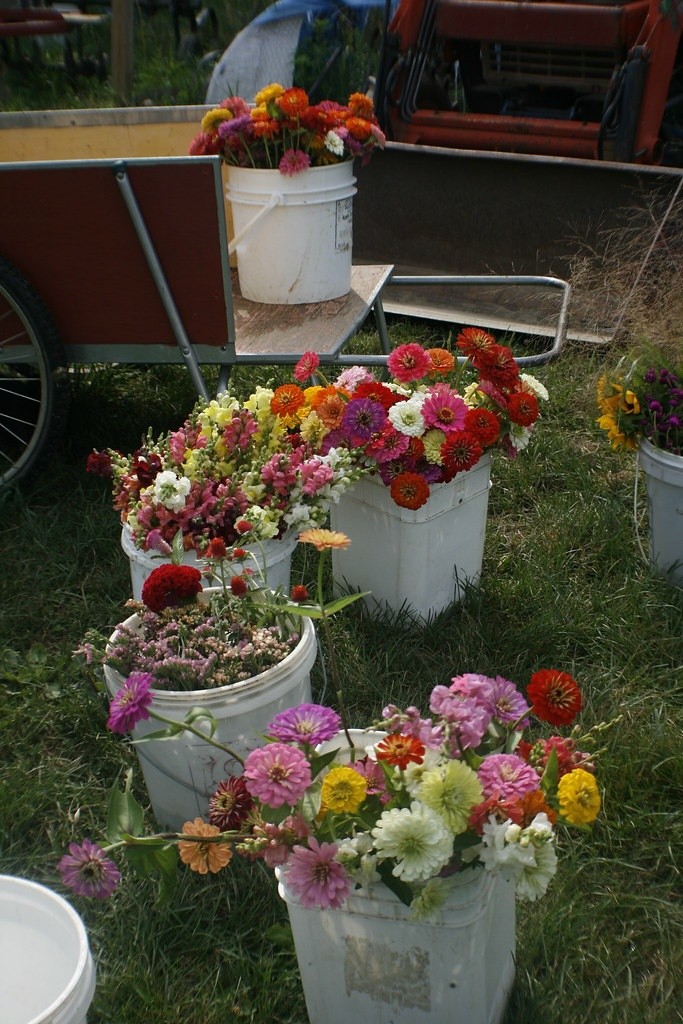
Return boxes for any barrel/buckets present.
[120,525,298,602]
[0,874,96,1024]
[634,436,683,581]
[103,587,326,834]
[221,158,357,304]
[330,452,492,631]
[274,852,516,1024]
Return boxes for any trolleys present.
[0,96,576,504]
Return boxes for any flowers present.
[57,527,623,923]
[84,326,549,560]
[189,76,385,179]
[596,336,683,459]
